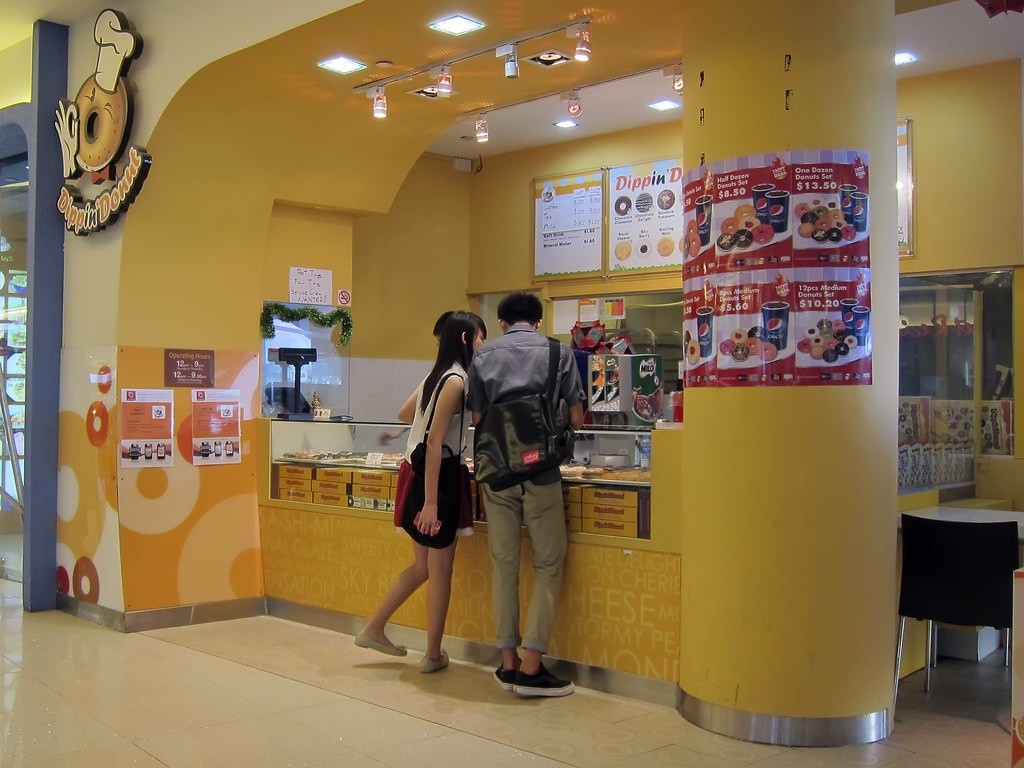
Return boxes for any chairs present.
[894,513,1019,707]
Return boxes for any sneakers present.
[493,662,515,690]
[513,661,574,696]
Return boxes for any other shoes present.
[419,647,449,673]
[355,632,408,657]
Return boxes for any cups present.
[751,184,776,224]
[696,306,714,358]
[761,301,790,350]
[838,184,858,225]
[840,298,859,337]
[851,305,870,346]
[765,190,791,233]
[850,192,868,232]
[695,194,713,246]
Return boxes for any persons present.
[466,293,587,696]
[355,310,487,671]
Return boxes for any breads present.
[615,237,675,260]
[282,452,404,462]
[636,189,676,213]
[558,462,650,482]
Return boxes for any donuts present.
[720,326,778,362]
[614,195,631,216]
[716,203,774,252]
[794,198,857,244]
[683,220,701,257]
[797,319,858,362]
[683,329,700,365]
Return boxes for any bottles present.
[641,435,651,472]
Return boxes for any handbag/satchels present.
[475,392,571,491]
[401,442,466,549]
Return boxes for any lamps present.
[476,118,488,143]
[505,52,520,78]
[672,71,683,95]
[437,65,452,98]
[575,33,592,62]
[568,90,583,117]
[373,85,387,118]
